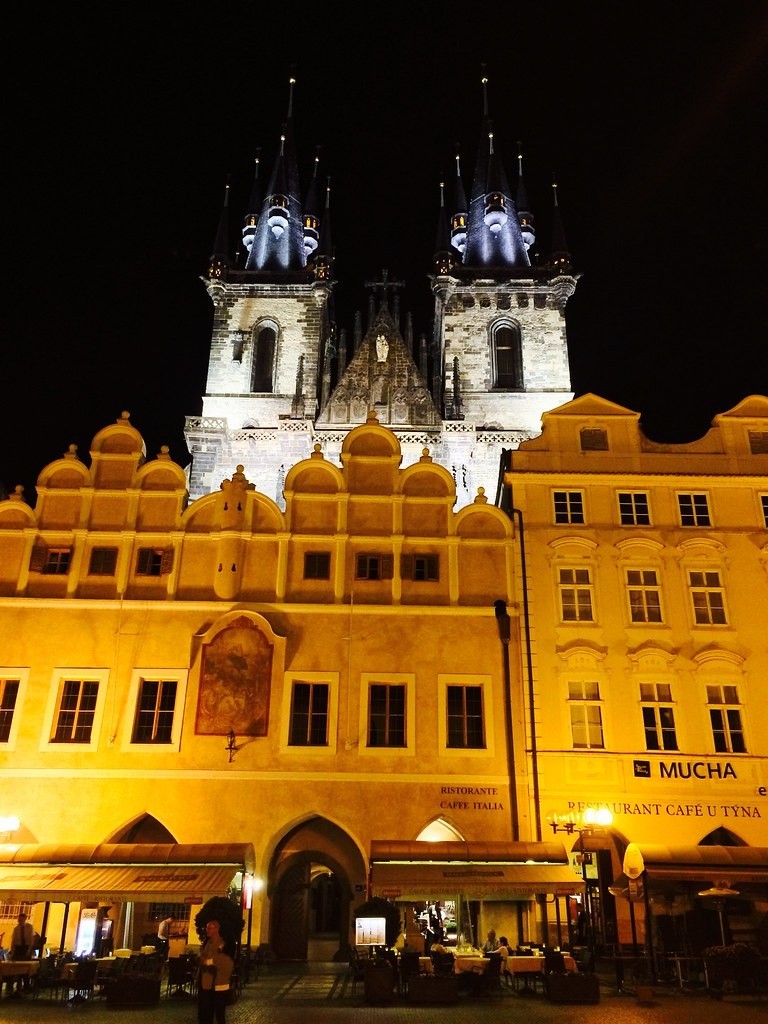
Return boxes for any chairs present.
[231,944,260,998]
[0,937,198,1006]
[344,941,595,998]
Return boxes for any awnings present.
[623,842,768,883]
[0,843,256,903]
[370,837,586,898]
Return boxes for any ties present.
[21,926,24,945]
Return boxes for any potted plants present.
[706,942,765,989]
[545,968,600,1000]
[106,973,161,1006]
[407,973,457,1003]
[364,956,395,999]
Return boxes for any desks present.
[536,951,570,957]
[96,956,130,996]
[61,962,87,1003]
[164,958,188,998]
[453,958,490,976]
[396,957,433,983]
[0,961,39,1000]
[445,945,484,957]
[505,956,579,997]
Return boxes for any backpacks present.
[33,932,47,950]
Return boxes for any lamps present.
[223,729,239,764]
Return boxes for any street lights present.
[546,808,614,973]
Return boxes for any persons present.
[198,920,233,1024]
[429,931,452,953]
[157,915,175,961]
[481,929,509,974]
[573,904,585,924]
[10,914,35,995]
